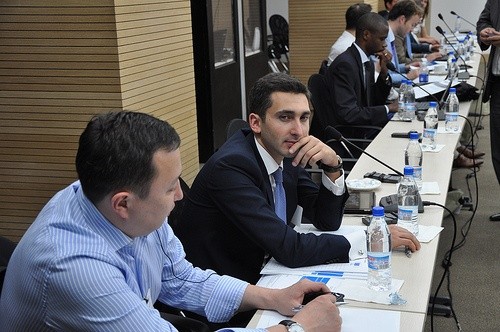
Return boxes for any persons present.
[476,0,500,221]
[163,74,422,328]
[379,0,487,166]
[327,3,400,100]
[0,110,342,332]
[311,11,398,151]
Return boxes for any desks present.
[246,34,492,332]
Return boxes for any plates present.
[346,178,381,190]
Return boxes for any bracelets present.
[316,155,343,173]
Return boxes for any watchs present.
[279,320,304,332]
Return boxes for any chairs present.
[307,74,381,173]
[262,13,289,74]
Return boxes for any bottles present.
[444,88,460,131]
[405,133,423,190]
[366,206,391,291]
[418,58,428,85]
[397,166,419,235]
[455,16,462,33]
[422,102,439,150]
[398,80,416,120]
[448,58,459,86]
[448,51,456,65]
[458,30,474,62]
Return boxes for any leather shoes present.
[452,154,484,168]
[458,144,485,158]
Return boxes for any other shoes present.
[490,213,500,221]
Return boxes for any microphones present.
[438,13,469,59]
[387,62,446,121]
[450,11,476,28]
[325,125,424,214]
[435,26,471,78]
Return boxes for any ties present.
[271,168,286,225]
[406,34,413,60]
[364,61,372,108]
[391,41,398,69]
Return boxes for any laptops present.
[412,81,453,111]
